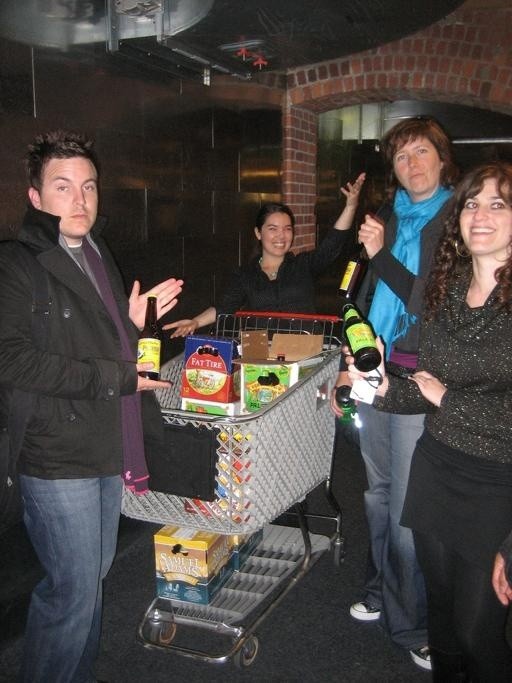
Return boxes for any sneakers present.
[349,598,381,622]
[411,644,432,671]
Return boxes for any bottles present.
[136,294,162,381]
[336,385,357,426]
[342,302,382,372]
[335,242,370,303]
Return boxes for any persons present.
[1,128,187,683]
[338,158,512,681]
[487,528,512,609]
[159,169,366,530]
[327,116,458,672]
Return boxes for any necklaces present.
[257,255,278,279]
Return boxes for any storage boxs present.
[153,523,264,608]
[180,328,343,522]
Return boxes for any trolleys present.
[121,312,346,671]
